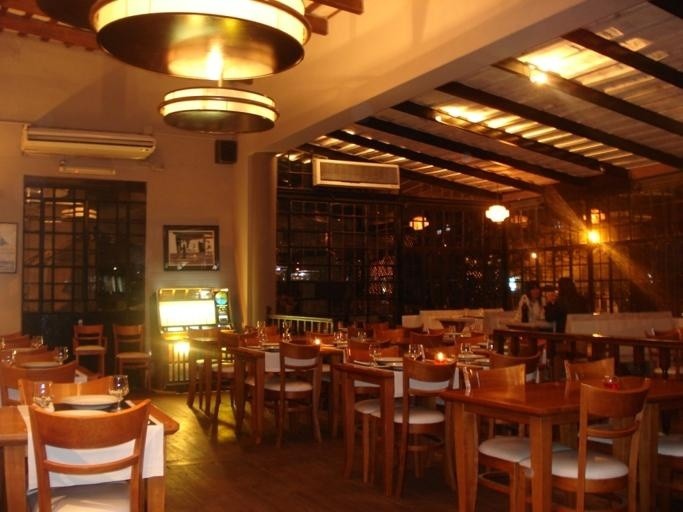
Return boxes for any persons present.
[513,280,544,321]
[544,274,587,331]
[540,284,558,319]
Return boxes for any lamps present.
[86,0,312,79]
[160,77,280,137]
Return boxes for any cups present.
[603,373,623,388]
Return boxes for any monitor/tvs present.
[159,301,217,327]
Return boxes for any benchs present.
[402,307,681,364]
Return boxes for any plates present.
[62,395,119,409]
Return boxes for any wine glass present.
[255,320,472,366]
[33,381,52,409]
[0,335,69,368]
[109,375,129,413]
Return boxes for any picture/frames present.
[162,224,220,272]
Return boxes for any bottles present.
[521,296,528,322]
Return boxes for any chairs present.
[441,326,682,512]
[1,322,180,512]
[189,330,347,448]
[346,323,442,499]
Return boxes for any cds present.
[215,291,228,305]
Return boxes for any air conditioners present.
[312,158,401,191]
[20,123,156,162]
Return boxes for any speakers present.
[214,139,236,164]
